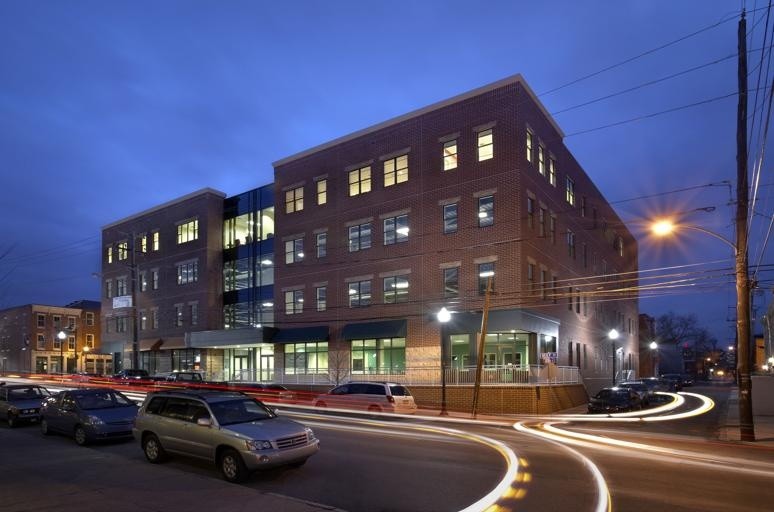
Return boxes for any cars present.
[240,383,295,402]
[587,372,697,414]
[67,372,108,385]
[0,381,52,427]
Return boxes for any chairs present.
[234,239,241,247]
[267,233,272,239]
[246,236,252,243]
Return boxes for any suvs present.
[133,389,322,482]
[310,379,418,417]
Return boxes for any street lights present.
[57,330,66,378]
[649,216,756,443]
[437,306,452,415]
[80,345,89,372]
[608,328,622,386]
[649,340,658,379]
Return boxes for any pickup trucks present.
[110,368,166,388]
[164,372,229,390]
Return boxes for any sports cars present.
[40,387,144,445]
